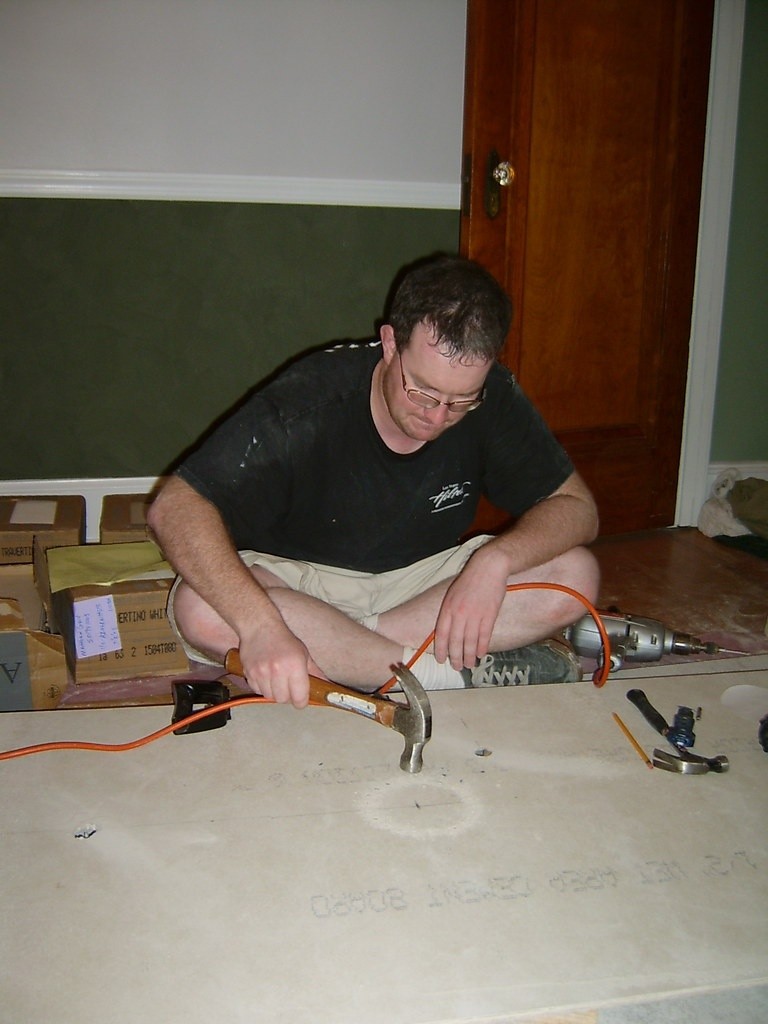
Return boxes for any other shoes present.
[459,638,584,690]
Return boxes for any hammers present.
[626,687,730,775]
[224,647,434,774]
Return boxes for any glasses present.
[399,345,485,412]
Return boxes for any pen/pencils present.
[609,712,654,769]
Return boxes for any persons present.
[141,251,600,712]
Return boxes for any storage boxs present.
[34,534,193,685]
[0,598,34,712]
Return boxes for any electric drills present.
[558,607,753,673]
